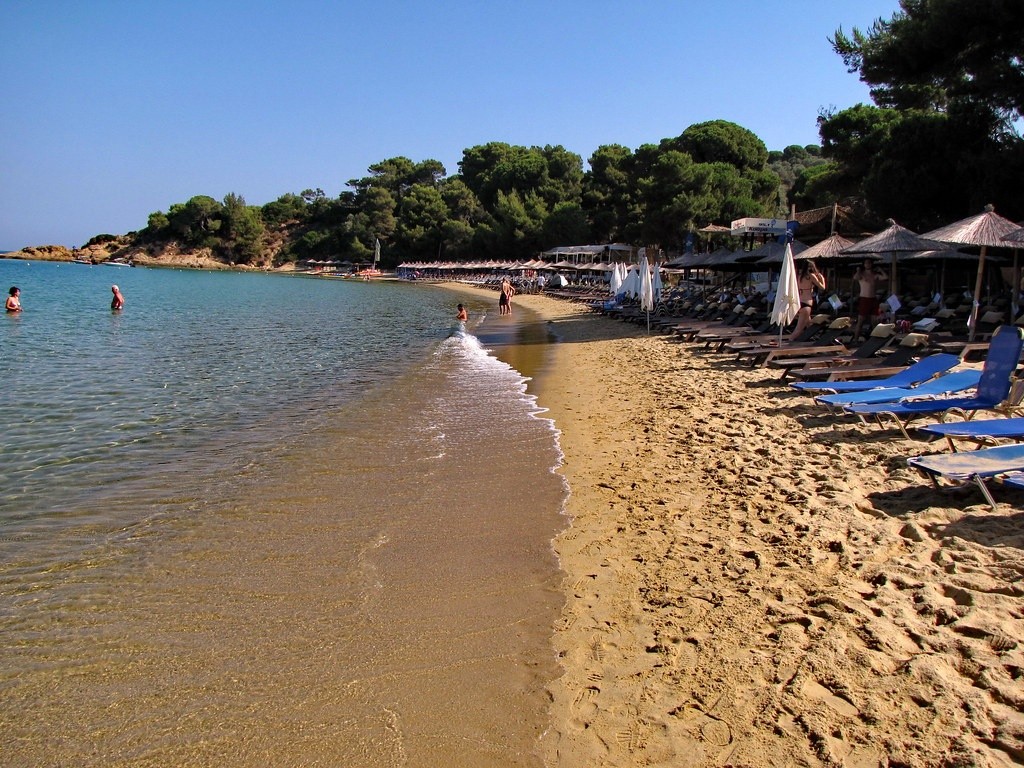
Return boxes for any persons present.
[456,303,467,322]
[788,259,825,342]
[111,285,124,310]
[361,268,741,317]
[853,258,890,344]
[5,287,24,313]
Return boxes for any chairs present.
[546,283,1024,509]
[479,278,522,295]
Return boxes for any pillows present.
[908,301,921,307]
[912,306,927,314]
[708,303,717,308]
[733,304,744,313]
[871,323,896,337]
[718,303,728,310]
[957,305,970,314]
[994,299,1008,307]
[682,302,691,308]
[695,305,704,311]
[900,333,930,346]
[829,317,852,328]
[744,307,757,315]
[982,306,997,313]
[811,314,830,324]
[981,311,1004,322]
[904,296,912,302]
[936,309,956,317]
[912,318,936,326]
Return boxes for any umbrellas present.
[306,257,352,266]
[397,203,1024,340]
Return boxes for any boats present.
[71,257,132,268]
